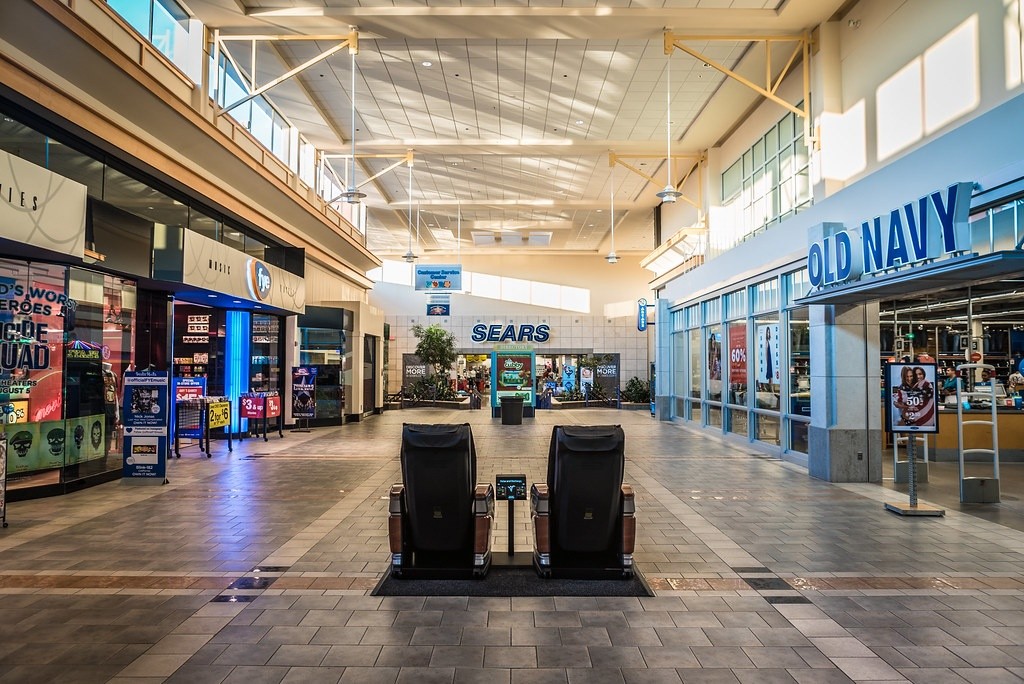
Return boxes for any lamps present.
[656,53,682,204]
[339,47,367,205]
[603,165,621,264]
[402,161,420,262]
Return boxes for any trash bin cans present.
[498,396,525,425]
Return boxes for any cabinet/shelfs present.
[181,313,279,347]
[880,353,1011,400]
[174,352,280,428]
[789,355,810,390]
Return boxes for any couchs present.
[387,421,495,580]
[529,423,637,581]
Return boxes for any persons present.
[763,326,774,383]
[893,366,935,426]
[543,368,552,380]
[939,367,964,401]
[461,365,483,395]
[981,370,993,386]
[710,333,721,380]
[1009,365,1024,391]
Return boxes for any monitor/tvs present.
[496,477,526,499]
